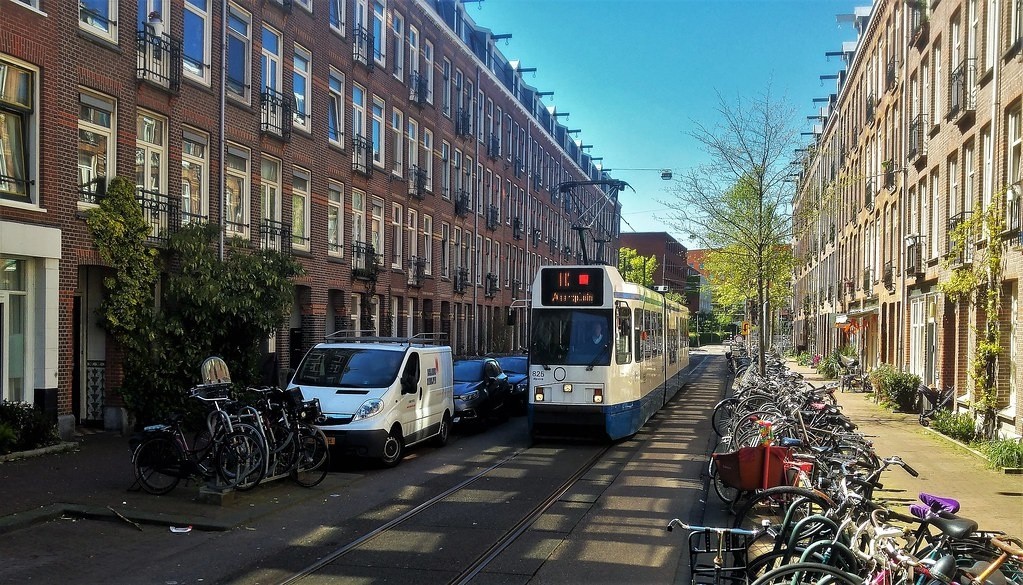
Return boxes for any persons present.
[584,323,610,356]
[373,352,403,386]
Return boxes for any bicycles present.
[666,334,1023,585]
[132,380,331,495]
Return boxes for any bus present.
[507,265,690,440]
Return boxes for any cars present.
[453,354,508,430]
[487,352,529,410]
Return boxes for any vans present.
[287,330,453,467]
